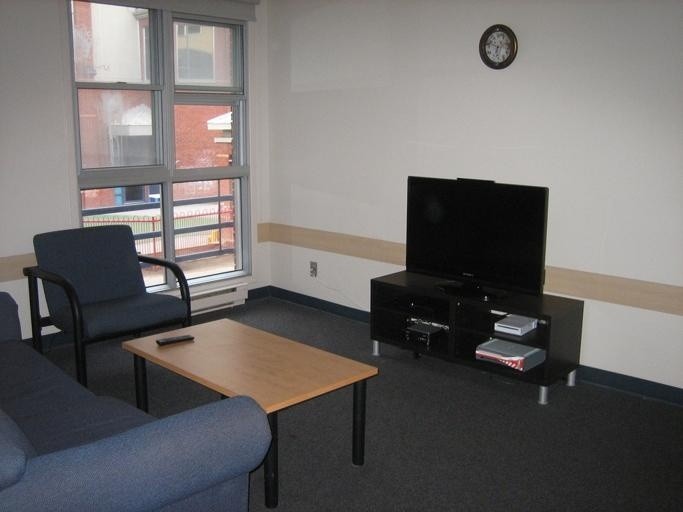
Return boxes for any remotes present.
[157,335,194,345]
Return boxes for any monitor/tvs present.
[406,176,549,302]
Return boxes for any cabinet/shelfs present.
[370,271,584,407]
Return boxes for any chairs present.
[23,224,191,389]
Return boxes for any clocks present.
[478,24,518,70]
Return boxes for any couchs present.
[1,292,272,512]
